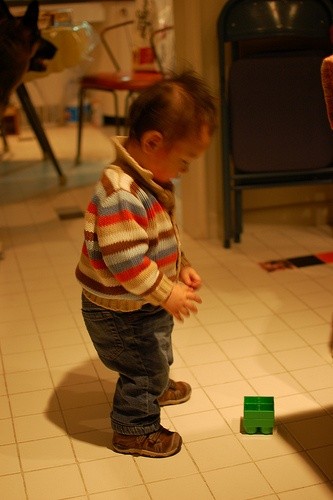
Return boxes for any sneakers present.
[155,379,190,406]
[112,425,182,457]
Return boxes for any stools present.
[73,74,164,164]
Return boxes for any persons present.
[76,68,221,460]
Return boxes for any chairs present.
[216,0,333,248]
[0,3,68,189]
